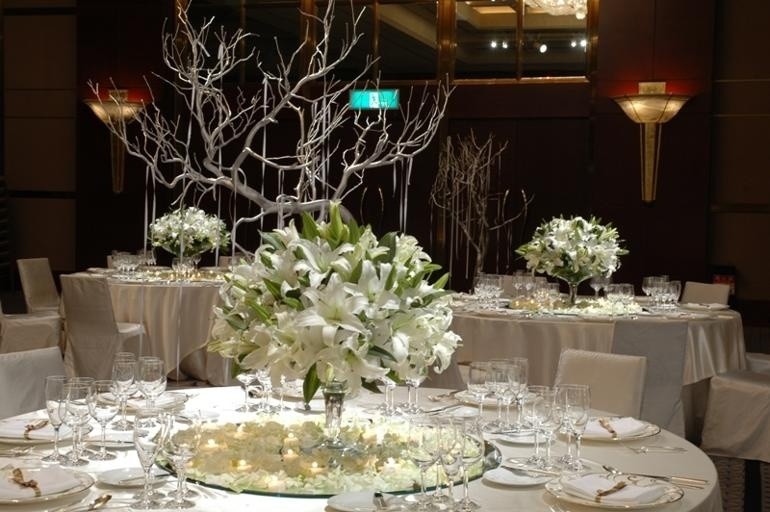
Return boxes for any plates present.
[273,378,323,399]
[544,415,683,508]
[0,418,94,506]
[327,491,397,512]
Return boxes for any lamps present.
[612,79,692,202]
[83,89,149,193]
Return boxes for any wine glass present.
[44,352,199,510]
[87,251,228,286]
[454,272,730,320]
[375,357,589,511]
[236,365,283,415]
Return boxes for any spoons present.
[602,464,709,486]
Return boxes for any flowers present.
[514,214,629,288]
[146,205,236,256]
[157,422,490,496]
[208,206,463,409]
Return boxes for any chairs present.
[698,372,770,462]
[1,256,747,440]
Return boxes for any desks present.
[3,383,722,512]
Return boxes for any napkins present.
[563,474,669,503]
[1,467,82,499]
